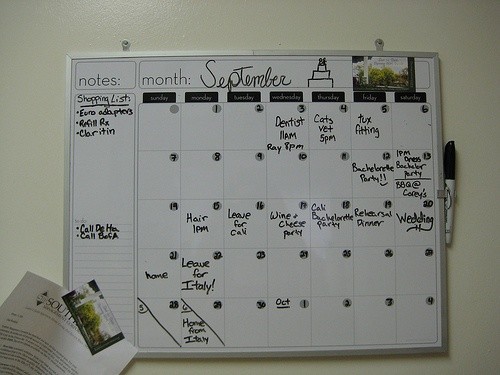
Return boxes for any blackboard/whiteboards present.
[59,49,449,359]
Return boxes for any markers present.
[443,141,456,245]
